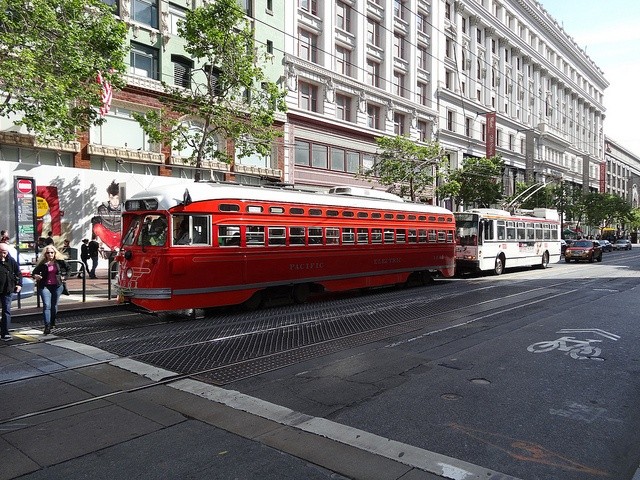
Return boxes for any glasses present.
[46,252,54,254]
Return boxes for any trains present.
[117,186,456,320]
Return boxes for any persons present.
[63,238,72,278]
[76,239,92,279]
[88,235,102,279]
[44,230,55,247]
[31,245,72,335]
[169,219,204,247]
[0,242,23,342]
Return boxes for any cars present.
[561,239,568,255]
[612,239,633,250]
[0,242,35,303]
[565,240,602,262]
[599,240,613,252]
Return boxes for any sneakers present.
[44,325,51,334]
[51,322,55,328]
[1,335,13,341]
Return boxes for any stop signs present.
[17,179,33,193]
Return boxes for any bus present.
[451,207,562,274]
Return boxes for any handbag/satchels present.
[55,261,70,295]
